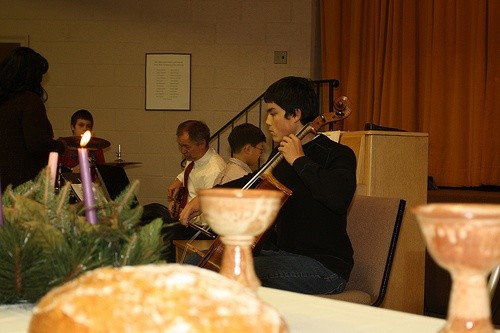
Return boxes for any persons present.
[178,75,357,295]
[58,109,106,184]
[163,120,228,240]
[0,46,56,194]
[211,122,267,186]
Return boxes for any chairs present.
[315,193,407,307]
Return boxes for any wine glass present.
[410,202,500,333]
[196,188,290,290]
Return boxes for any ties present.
[179,161,195,218]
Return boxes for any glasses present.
[250,145,265,153]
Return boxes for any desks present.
[338,130,429,316]
[0,286,447,333]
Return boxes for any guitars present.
[170,187,188,218]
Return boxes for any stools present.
[172,237,216,263]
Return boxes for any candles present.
[78,130,98,226]
[45,152,58,199]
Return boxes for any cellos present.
[179,96,352,273]
